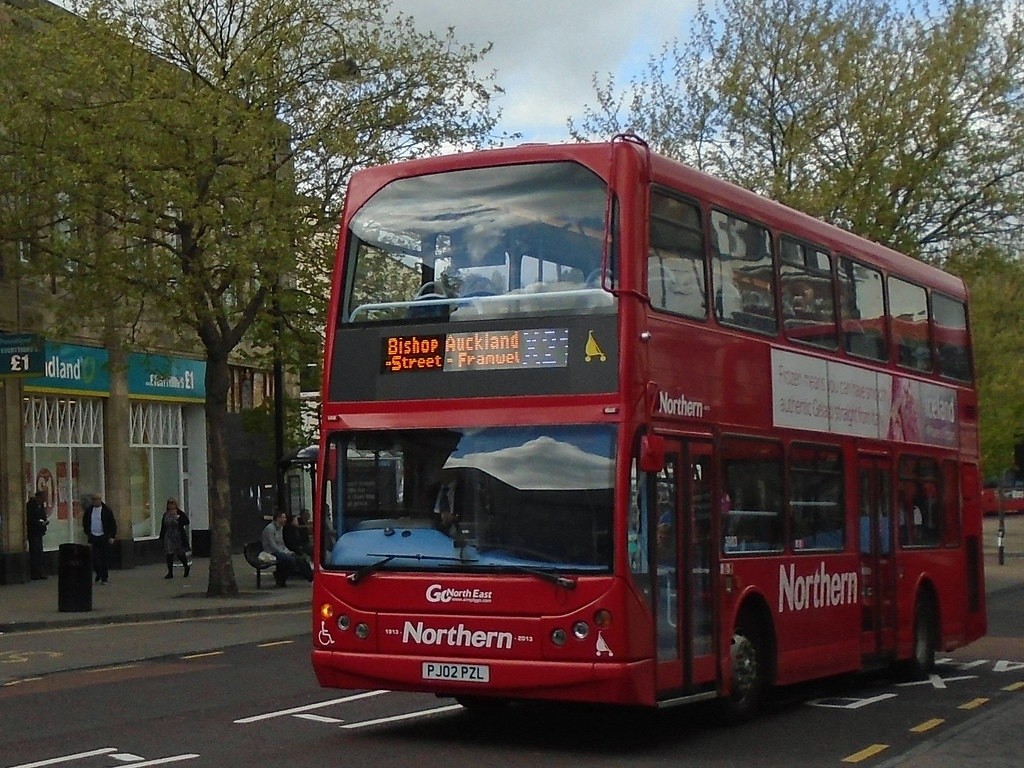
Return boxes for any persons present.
[26,490,50,580]
[82,493,117,584]
[298,509,312,543]
[323,504,336,546]
[282,515,314,583]
[160,498,191,578]
[262,511,296,588]
[421,459,475,537]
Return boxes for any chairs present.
[732,307,907,364]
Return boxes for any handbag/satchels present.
[258,551,276,564]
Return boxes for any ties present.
[439,486,451,516]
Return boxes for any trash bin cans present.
[58,543,92,613]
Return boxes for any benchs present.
[244,540,280,586]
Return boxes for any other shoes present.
[95,575,101,583]
[102,579,108,584]
[32,574,48,580]
[184,566,190,578]
[164,572,174,579]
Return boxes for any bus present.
[978,462,1024,516]
[307,130,992,721]
